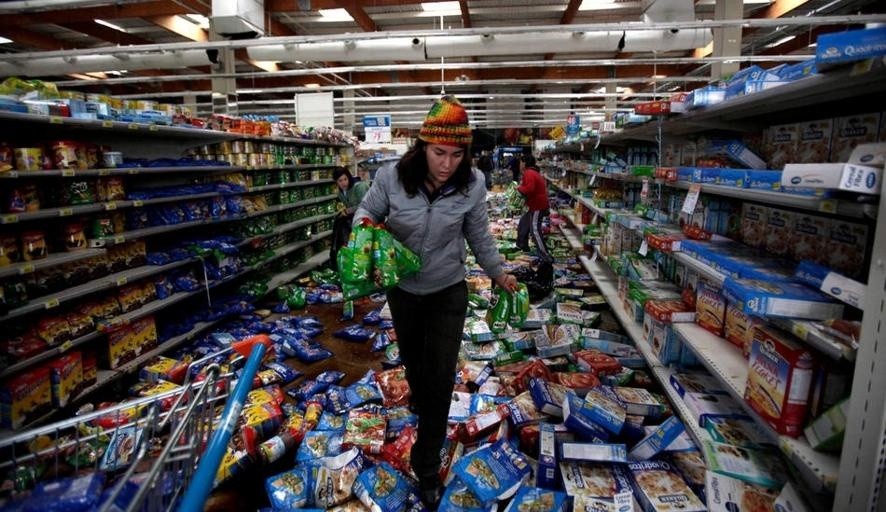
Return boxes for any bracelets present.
[501,275,510,288]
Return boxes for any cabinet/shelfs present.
[0,87,357,438]
[541,53,886,512]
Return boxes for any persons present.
[514,154,550,257]
[477,150,493,190]
[352,97,518,506]
[334,167,371,216]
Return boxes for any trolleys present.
[0,330,271,512]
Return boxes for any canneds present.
[186,140,267,166]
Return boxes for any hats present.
[418,95,473,146]
